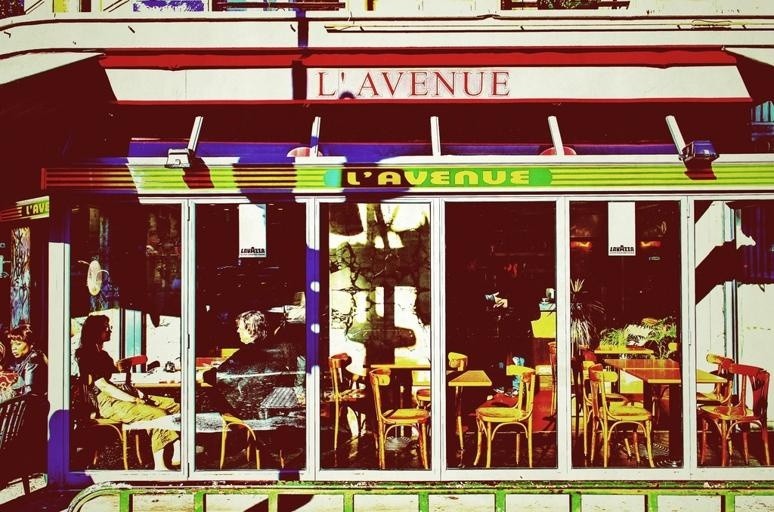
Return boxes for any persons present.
[73,311,181,470]
[195,306,287,420]
[4,324,48,398]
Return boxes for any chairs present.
[69,347,432,472]
[449,352,773,470]
[1,393,50,503]
